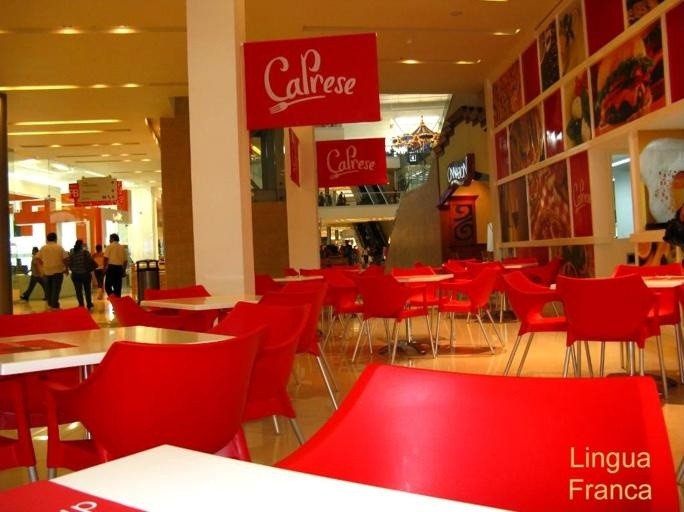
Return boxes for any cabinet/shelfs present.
[483,0,684,316]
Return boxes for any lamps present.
[44,148,55,214]
[12,148,21,213]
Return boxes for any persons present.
[33,232,68,310]
[68,244,98,309]
[319,191,324,206]
[389,145,393,156]
[19,246,49,300]
[319,238,377,266]
[338,191,345,203]
[90,243,105,300]
[69,240,82,255]
[324,191,332,207]
[102,233,127,310]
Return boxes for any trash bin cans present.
[137,259,160,304]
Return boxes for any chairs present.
[0,253,684,512]
